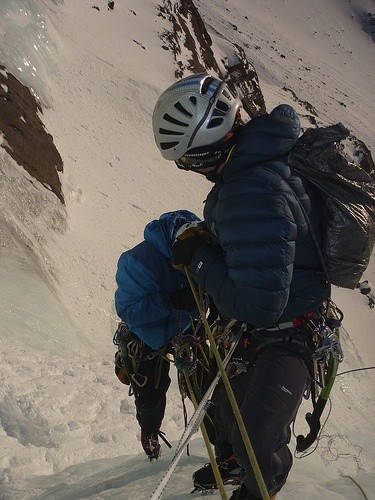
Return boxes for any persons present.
[114,210,218,462]
[152,73,332,500]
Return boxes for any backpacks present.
[286,121,375,290]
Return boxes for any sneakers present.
[228,482,277,500]
[140,430,161,458]
[188,459,244,494]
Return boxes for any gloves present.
[171,222,213,266]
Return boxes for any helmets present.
[151,72,244,161]
